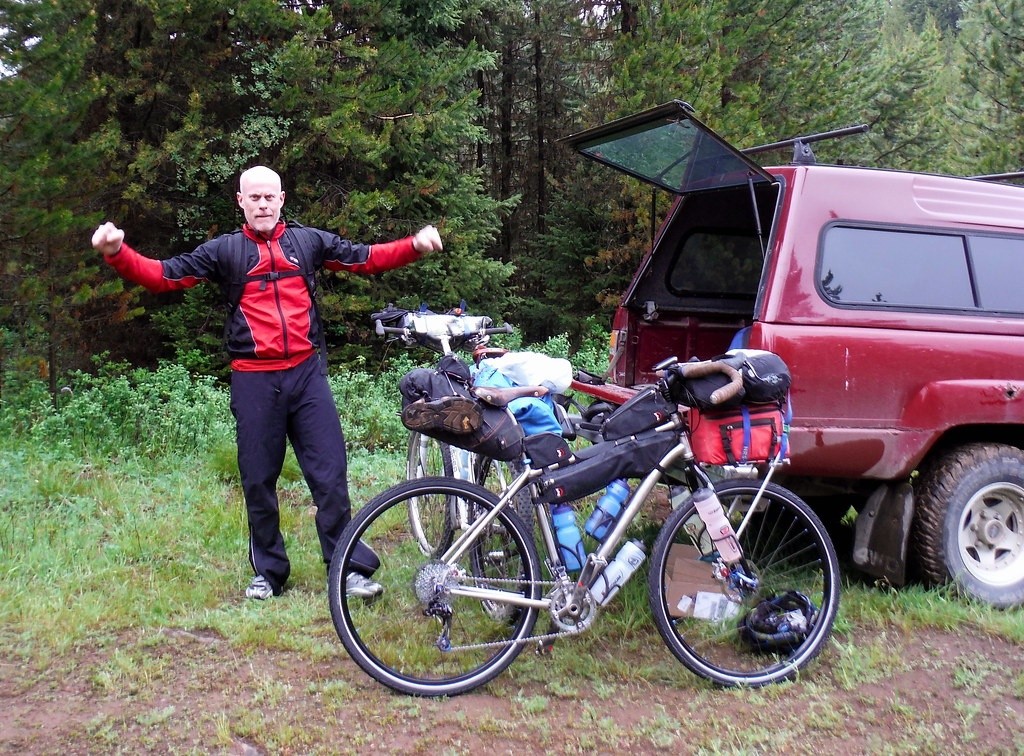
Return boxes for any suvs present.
[556,99,1024,613]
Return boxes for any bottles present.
[591,538,647,608]
[667,483,716,559]
[584,478,630,539]
[551,502,587,571]
[691,487,744,563]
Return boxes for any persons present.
[89,166,442,599]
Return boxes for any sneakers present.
[346,571,383,598]
[400,395,484,435]
[246,575,273,600]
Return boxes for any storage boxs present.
[664,543,740,620]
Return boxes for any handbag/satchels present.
[744,588,819,649]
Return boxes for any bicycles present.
[373,303,536,628]
[328,352,844,699]
